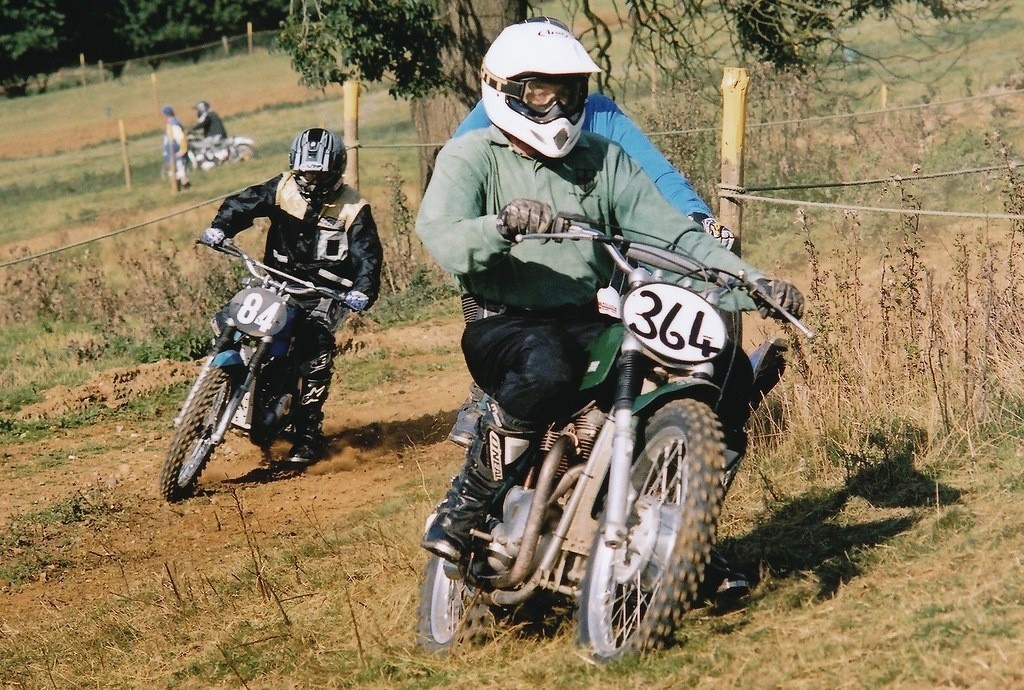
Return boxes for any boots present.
[419,396,544,563]
[286,378,330,465]
[448,380,492,448]
[698,431,751,615]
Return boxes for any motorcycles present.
[418,212,816,663]
[160,130,258,184]
[158,238,366,503]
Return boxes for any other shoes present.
[182,182,191,190]
[176,178,181,191]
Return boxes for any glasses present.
[481,61,583,115]
[290,172,344,186]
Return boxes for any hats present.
[163,106,173,115]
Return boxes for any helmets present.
[192,101,210,117]
[289,127,347,205]
[480,17,602,158]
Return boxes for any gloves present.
[496,199,553,245]
[344,290,370,312]
[201,227,225,248]
[688,212,736,251]
[752,279,804,323]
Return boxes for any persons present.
[200,129,383,461]
[189,102,227,164]
[445,17,785,446]
[415,22,804,597]
[161,106,190,189]
